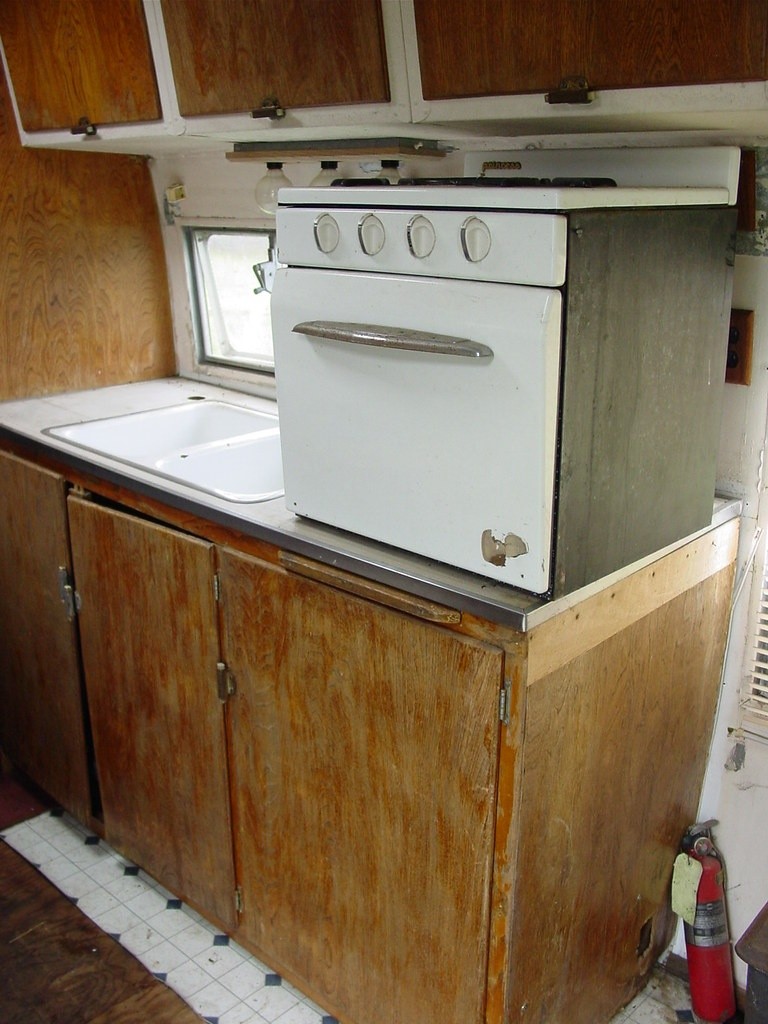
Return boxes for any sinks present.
[39,398,308,504]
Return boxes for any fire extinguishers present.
[678,819,739,1024]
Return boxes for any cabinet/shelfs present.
[0,374,737,1024]
